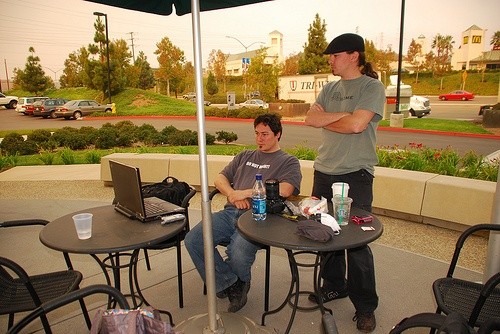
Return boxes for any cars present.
[238,100,269,110]
[183,92,212,106]
[247,91,260,97]
[55,99,112,120]
[438,89,475,101]
[21,101,37,116]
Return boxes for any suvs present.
[0,92,18,109]
[409,95,431,118]
[33,99,72,119]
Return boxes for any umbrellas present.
[89,0,279,334]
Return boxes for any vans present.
[16,97,49,115]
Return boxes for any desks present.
[237,199,384,334]
[39,205,186,327]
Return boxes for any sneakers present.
[352,311,376,332]
[308,278,349,304]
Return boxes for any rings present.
[230,196,231,198]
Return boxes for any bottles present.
[251,174,267,221]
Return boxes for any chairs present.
[387,224,500,334]
[0,185,196,334]
[209,184,270,312]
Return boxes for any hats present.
[322,33,365,55]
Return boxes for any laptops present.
[109,159,185,223]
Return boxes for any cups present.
[72,213,93,240]
[332,182,349,198]
[332,196,353,226]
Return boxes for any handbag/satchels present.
[141,176,190,207]
[91,306,166,334]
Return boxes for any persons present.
[274,87,279,99]
[184,114,302,314]
[305,34,387,333]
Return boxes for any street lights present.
[93,11,113,108]
[43,66,65,86]
[226,35,266,98]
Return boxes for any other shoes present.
[228,280,250,312]
